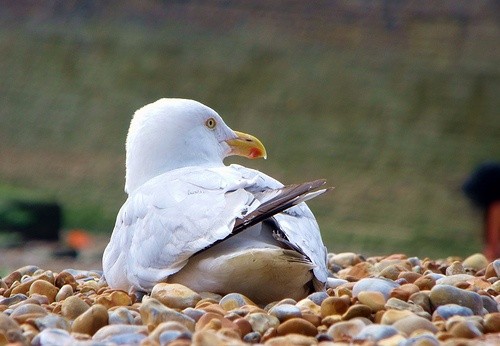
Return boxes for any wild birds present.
[98,97,336,308]
